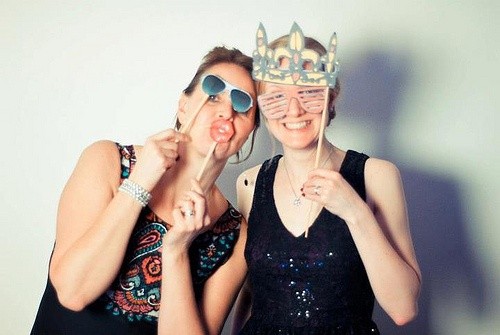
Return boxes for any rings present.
[314,186,320,194]
[184,210,194,215]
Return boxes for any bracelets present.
[118,179,152,206]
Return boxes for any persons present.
[220,34,422,335]
[30,45,260,335]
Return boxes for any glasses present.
[199,73,257,112]
[256,87,329,120]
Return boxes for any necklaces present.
[284,148,334,205]
